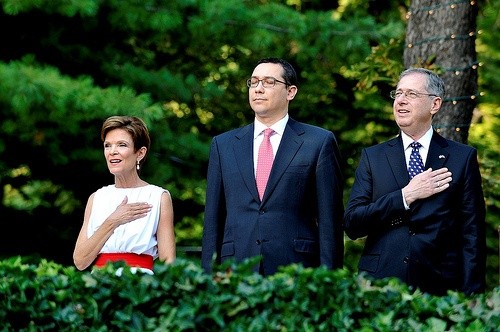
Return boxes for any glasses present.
[247,77,291,88]
[390,90,439,100]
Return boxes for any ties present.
[408,142,424,182]
[256,128,274,202]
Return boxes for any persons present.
[201,57,344,278]
[72,115,176,276]
[345,67,486,298]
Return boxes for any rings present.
[436,182,440,187]
